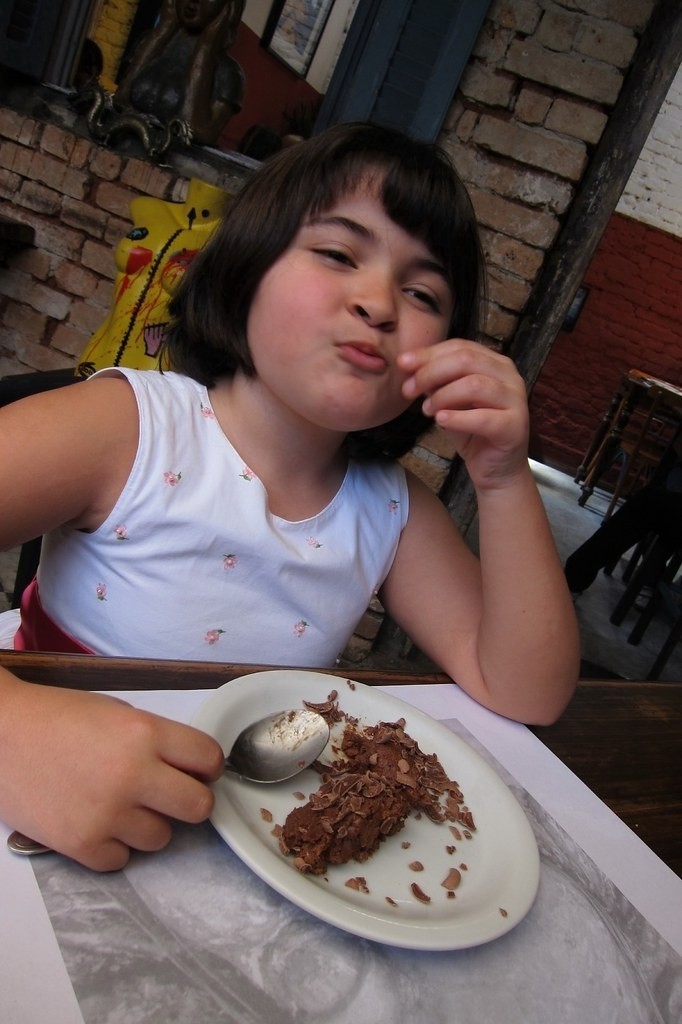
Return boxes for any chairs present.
[575,369,682,526]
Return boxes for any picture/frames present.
[258,0,336,81]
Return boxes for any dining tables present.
[0,647,682,1024]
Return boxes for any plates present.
[194,668,542,949]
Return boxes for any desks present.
[574,370,682,509]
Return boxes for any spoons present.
[6,710,331,855]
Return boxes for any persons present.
[0,122,582,875]
